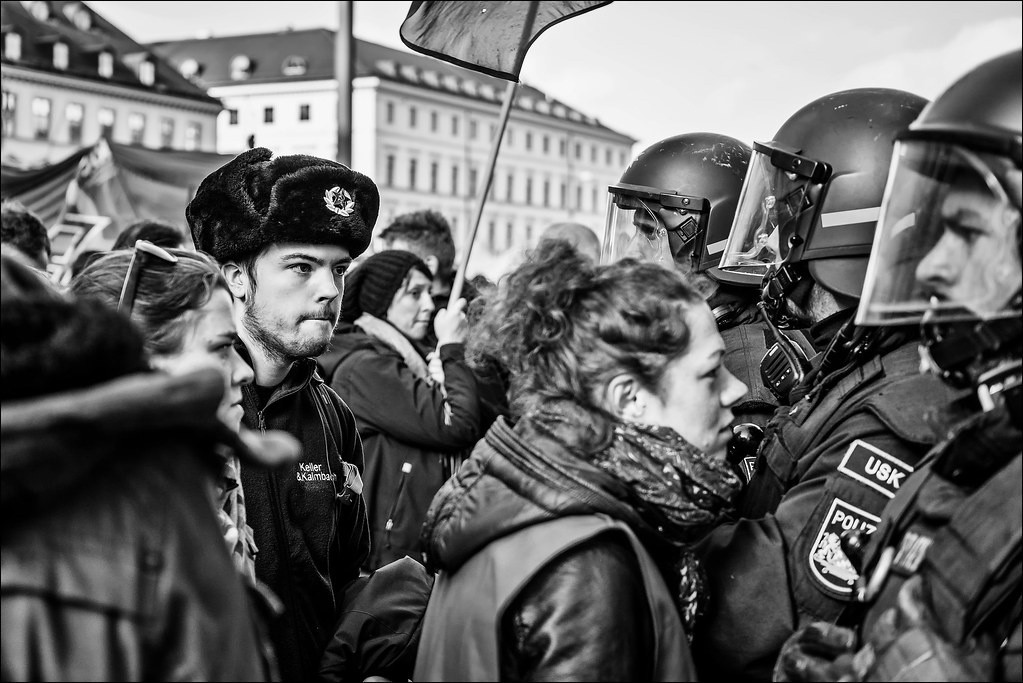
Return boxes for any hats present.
[185,146,380,265]
[339,249,423,319]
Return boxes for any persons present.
[0,294,271,683]
[409,238,750,683]
[0,52,1023,683]
[184,147,380,683]
[70,240,279,682]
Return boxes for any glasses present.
[115,238,178,320]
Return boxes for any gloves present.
[854,578,1005,683]
[767,619,858,683]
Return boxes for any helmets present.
[608,130,753,274]
[893,46,1023,311]
[752,86,933,303]
